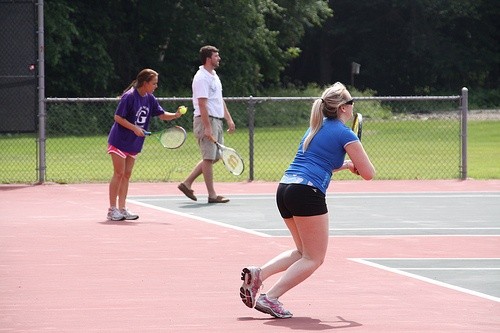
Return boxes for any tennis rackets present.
[144,125,186,149]
[352,112,363,175]
[211,136,245,176]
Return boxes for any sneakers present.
[178,183,197,201]
[240,267,263,308]
[106,208,126,221]
[118,208,139,220]
[208,196,229,203]
[254,294,293,319]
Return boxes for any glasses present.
[345,98,354,105]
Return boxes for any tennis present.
[179,106,187,114]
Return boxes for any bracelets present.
[175,113,177,119]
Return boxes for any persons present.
[106,69,183,221]
[239,81,375,319]
[177,46,235,203]
